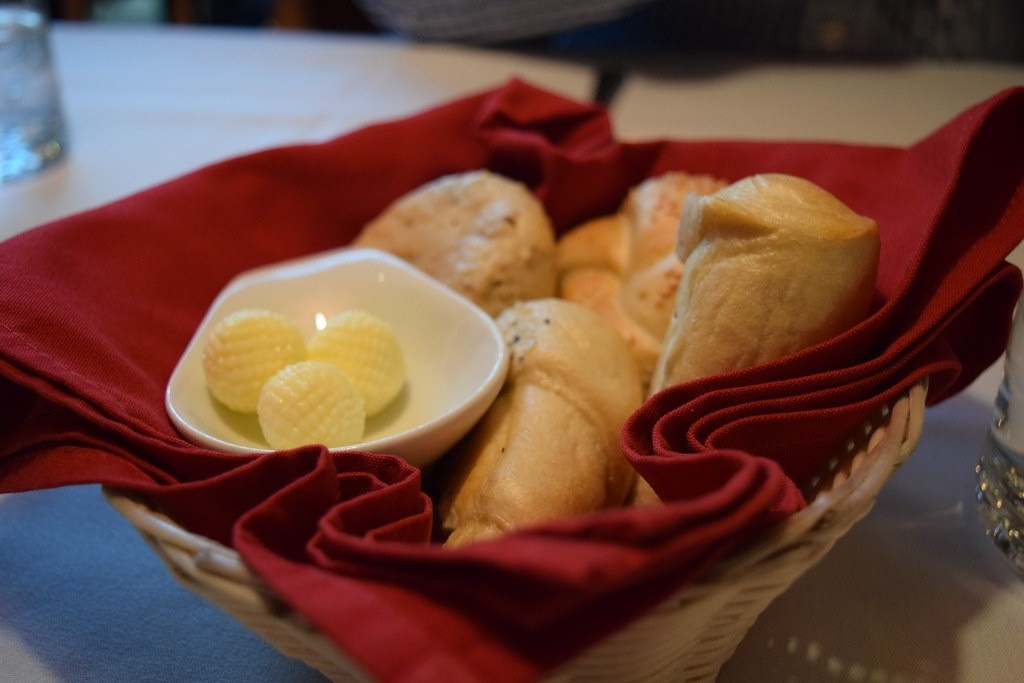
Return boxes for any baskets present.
[101,377,929,683]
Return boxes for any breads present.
[351,167,880,548]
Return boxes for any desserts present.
[199,306,401,450]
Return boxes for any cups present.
[0,1,69,179]
[975,291,1024,573]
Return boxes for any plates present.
[165,246,509,471]
